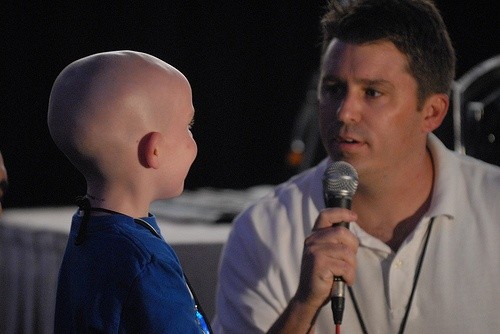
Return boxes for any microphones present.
[322,161,359,325]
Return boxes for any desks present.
[0,184,276,334]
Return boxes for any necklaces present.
[334,217,438,334]
[78,197,216,334]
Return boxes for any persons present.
[44,50,217,334]
[211,1,500,334]
